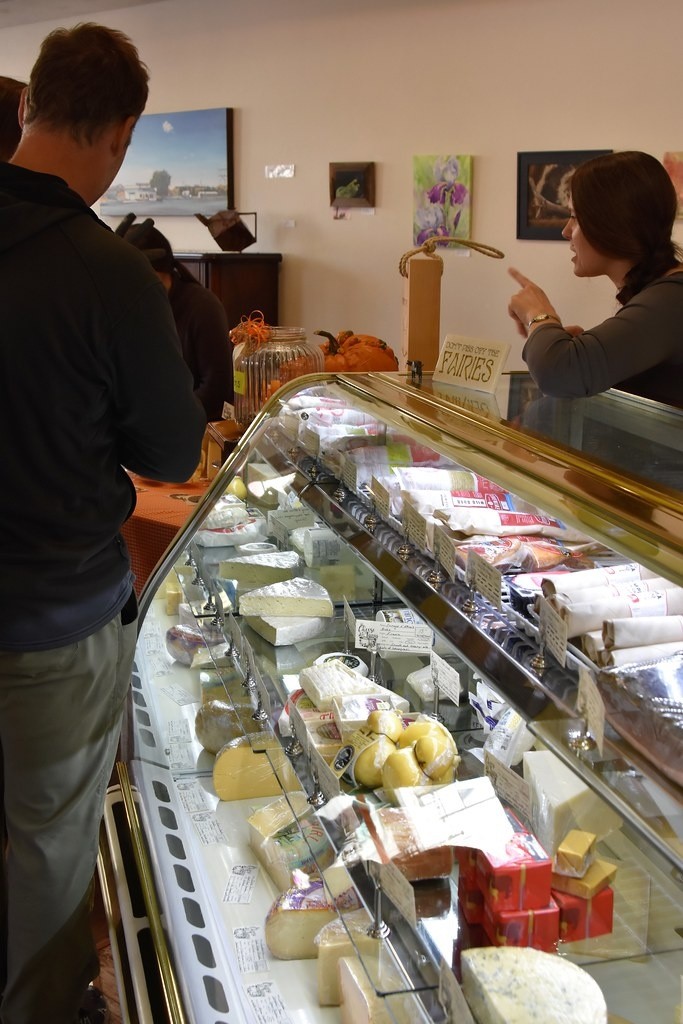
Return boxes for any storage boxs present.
[452,805,614,958]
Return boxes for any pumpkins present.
[320,330,398,372]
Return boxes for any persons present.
[509,149,683,499]
[0,20,235,1022]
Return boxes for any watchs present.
[528,311,562,330]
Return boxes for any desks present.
[120,469,230,602]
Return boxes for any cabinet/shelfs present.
[169,252,286,329]
[117,369,683,1024]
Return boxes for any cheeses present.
[163,460,617,1024]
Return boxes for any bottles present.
[232,326,325,432]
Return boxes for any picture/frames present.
[517,149,615,241]
[328,160,377,209]
